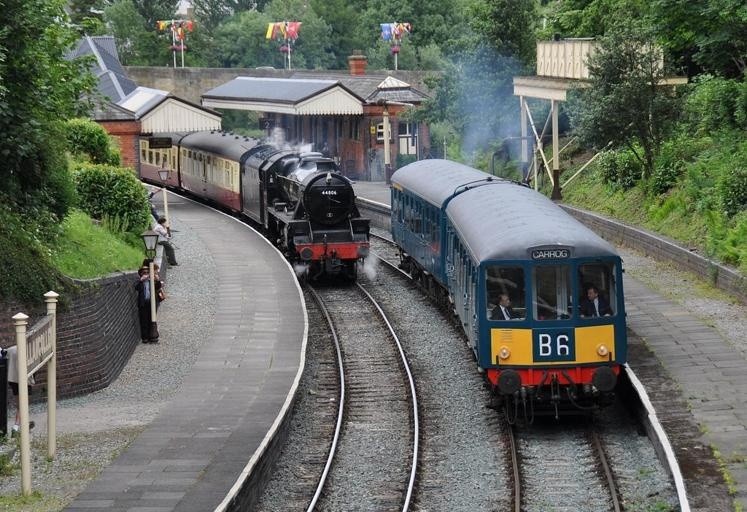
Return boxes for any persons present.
[132,266,161,345]
[154,264,166,307]
[7,343,36,436]
[578,284,613,321]
[154,217,180,266]
[492,292,520,321]
[151,204,160,220]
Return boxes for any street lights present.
[140,222,161,345]
[157,158,173,238]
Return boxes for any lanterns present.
[169,45,178,51]
[177,44,186,52]
[279,46,288,53]
[391,47,399,54]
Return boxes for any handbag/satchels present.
[159,288,166,301]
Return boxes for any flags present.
[177,19,192,36]
[156,19,172,31]
[380,22,392,43]
[265,22,283,41]
[285,22,302,40]
[394,22,410,44]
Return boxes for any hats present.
[138,266,149,275]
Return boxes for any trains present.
[386,155,629,435]
[138,129,373,290]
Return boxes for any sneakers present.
[142,337,159,344]
